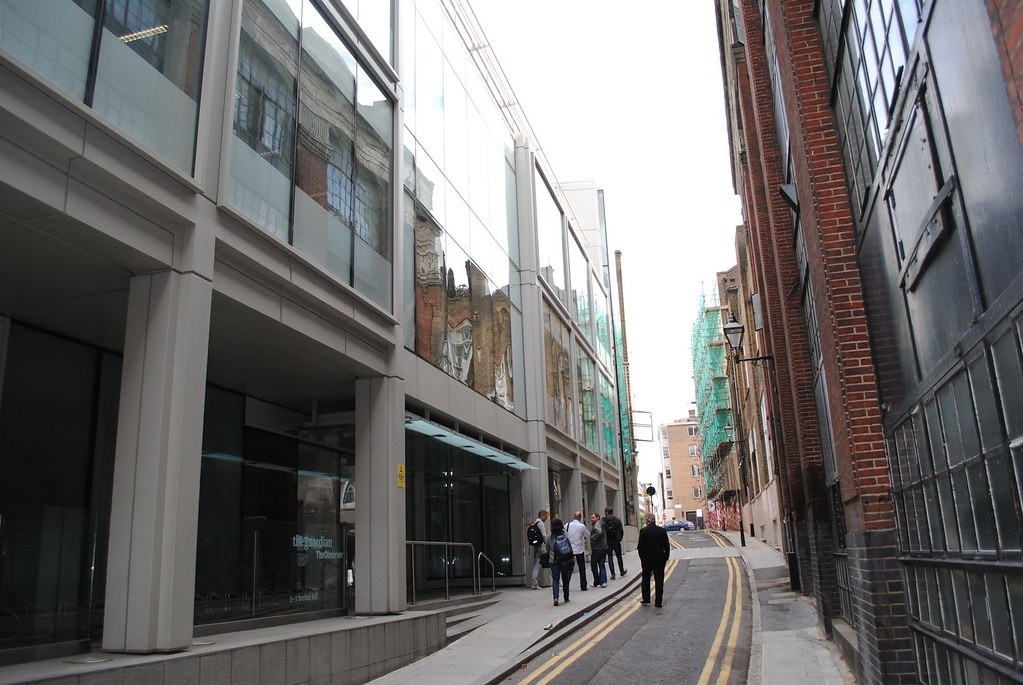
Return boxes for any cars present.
[662,517,694,531]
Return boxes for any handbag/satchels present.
[540,553,550,568]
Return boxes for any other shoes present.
[554,598,559,606]
[610,574,615,580]
[641,601,651,606]
[581,588,587,591]
[526,584,542,590]
[620,569,627,576]
[597,583,607,588]
[565,599,570,603]
[655,603,662,607]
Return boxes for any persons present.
[563,512,590,591]
[638,512,671,608]
[601,506,627,580]
[531,510,563,590]
[546,518,574,606]
[590,513,607,588]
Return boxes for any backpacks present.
[554,535,574,569]
[527,521,546,556]
[603,516,619,540]
